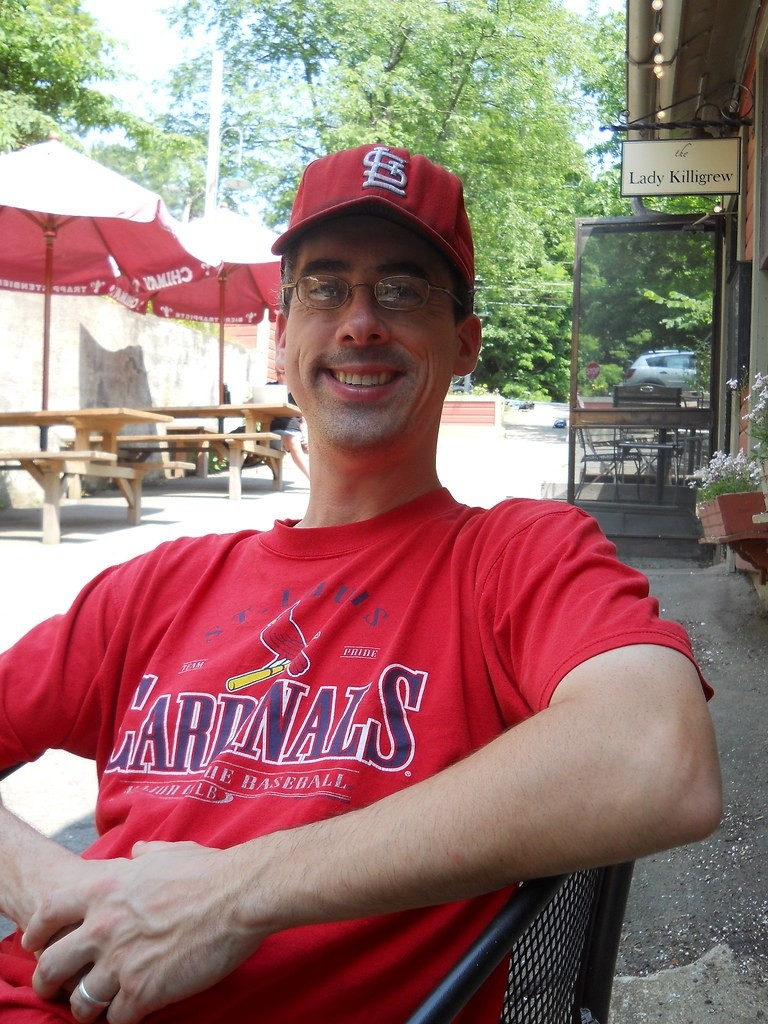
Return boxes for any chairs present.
[670,391,704,476]
[573,383,685,506]
[411,850,635,1024]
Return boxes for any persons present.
[0,144,724,1024]
[243,356,310,481]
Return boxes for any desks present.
[573,408,715,503]
[0,407,175,547]
[85,403,303,503]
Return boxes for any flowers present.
[685,364,768,515]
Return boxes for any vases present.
[697,492,765,537]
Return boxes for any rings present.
[78,975,111,1007]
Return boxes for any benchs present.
[0,431,295,473]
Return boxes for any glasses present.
[279,274,463,312]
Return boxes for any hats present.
[270,144,475,314]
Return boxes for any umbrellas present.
[141,207,282,404]
[0,131,218,451]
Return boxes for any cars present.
[623,350,697,391]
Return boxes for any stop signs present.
[586,362,600,381]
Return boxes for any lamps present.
[652,0,668,120]
